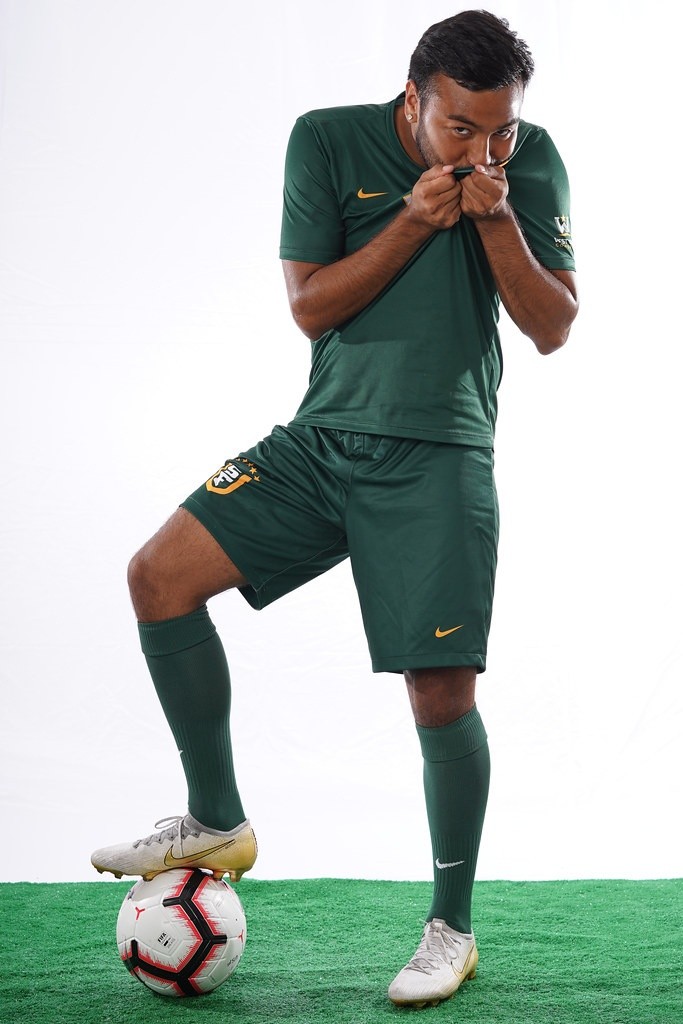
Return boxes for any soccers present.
[115,866,247,998]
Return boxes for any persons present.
[91,9,577,1010]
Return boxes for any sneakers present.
[89,814,259,882]
[388,925,478,1007]
